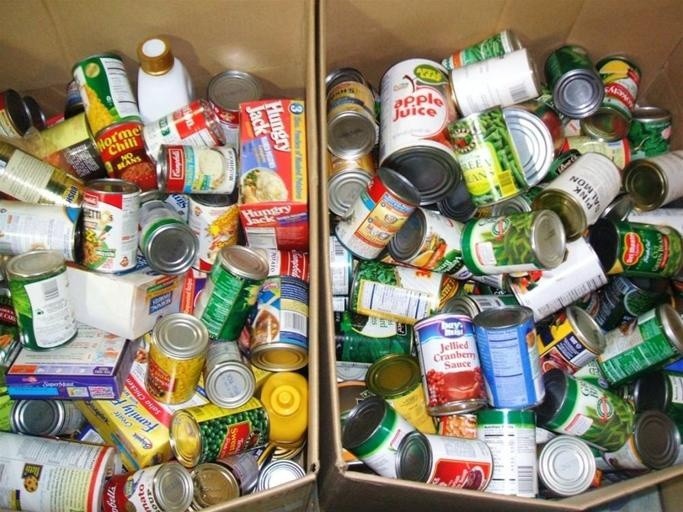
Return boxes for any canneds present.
[324,29,683,499]
[1,52,311,511]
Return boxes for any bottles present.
[137,37,193,121]
[259,370,309,449]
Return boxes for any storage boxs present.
[2,2,322,510]
[318,1,683,512]
